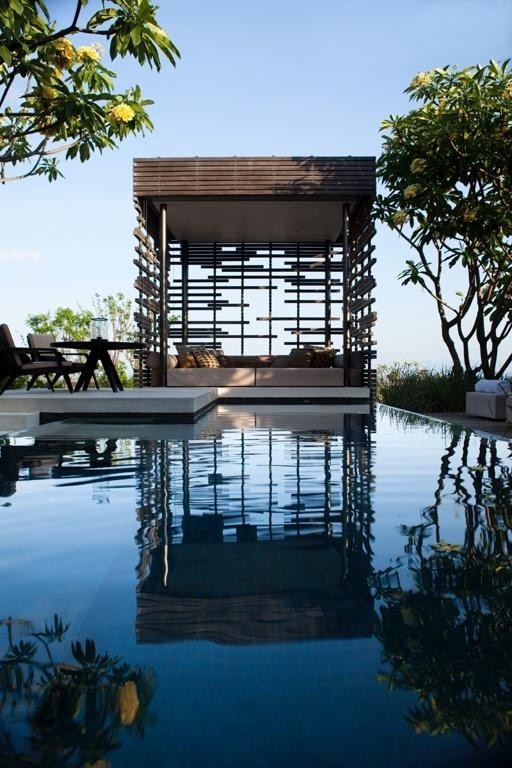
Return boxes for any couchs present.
[147,349,366,388]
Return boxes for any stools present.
[465,391,508,420]
[506,398,512,424]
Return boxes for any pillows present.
[175,345,204,367]
[303,344,325,353]
[310,346,343,368]
[192,346,223,370]
[286,347,315,369]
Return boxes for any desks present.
[46,334,151,396]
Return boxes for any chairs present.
[0,322,75,393]
[25,329,102,395]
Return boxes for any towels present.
[474,378,511,394]
